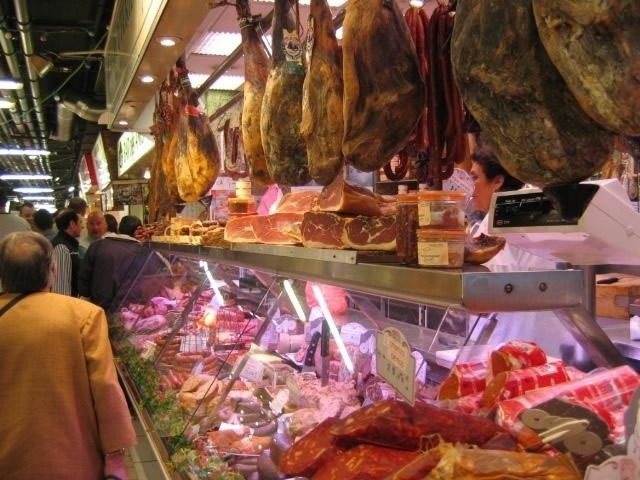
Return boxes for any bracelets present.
[105,447,128,458]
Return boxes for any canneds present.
[395,195,418,264]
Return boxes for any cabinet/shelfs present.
[107,240,634,479]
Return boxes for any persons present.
[1,230,139,479]
[464,151,582,374]
[1,176,161,312]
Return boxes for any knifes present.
[302,330,320,372]
[320,319,332,388]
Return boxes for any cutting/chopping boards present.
[593,272,640,319]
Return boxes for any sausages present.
[152,335,581,480]
